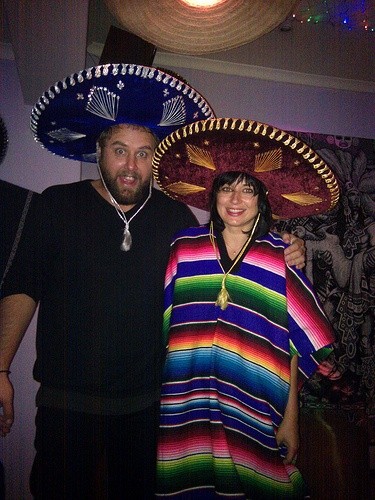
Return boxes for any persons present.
[0,64,306,500]
[152,118,340,500]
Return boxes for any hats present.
[151,117,341,219]
[29,63,218,163]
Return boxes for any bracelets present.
[0,370,11,374]
